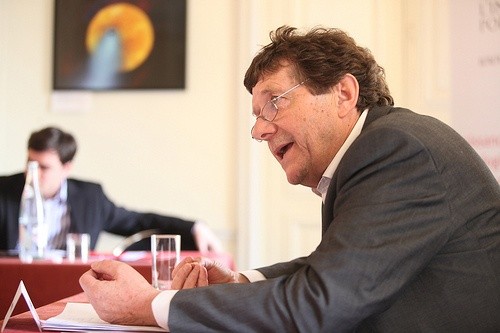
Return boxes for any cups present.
[151,234,181,291]
[66,233,90,263]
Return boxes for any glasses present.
[251,77,309,143]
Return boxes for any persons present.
[78,25,500,333]
[0,126,219,259]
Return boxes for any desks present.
[0,289,170,333]
[0,251,238,321]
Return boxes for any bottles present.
[18,161,45,260]
